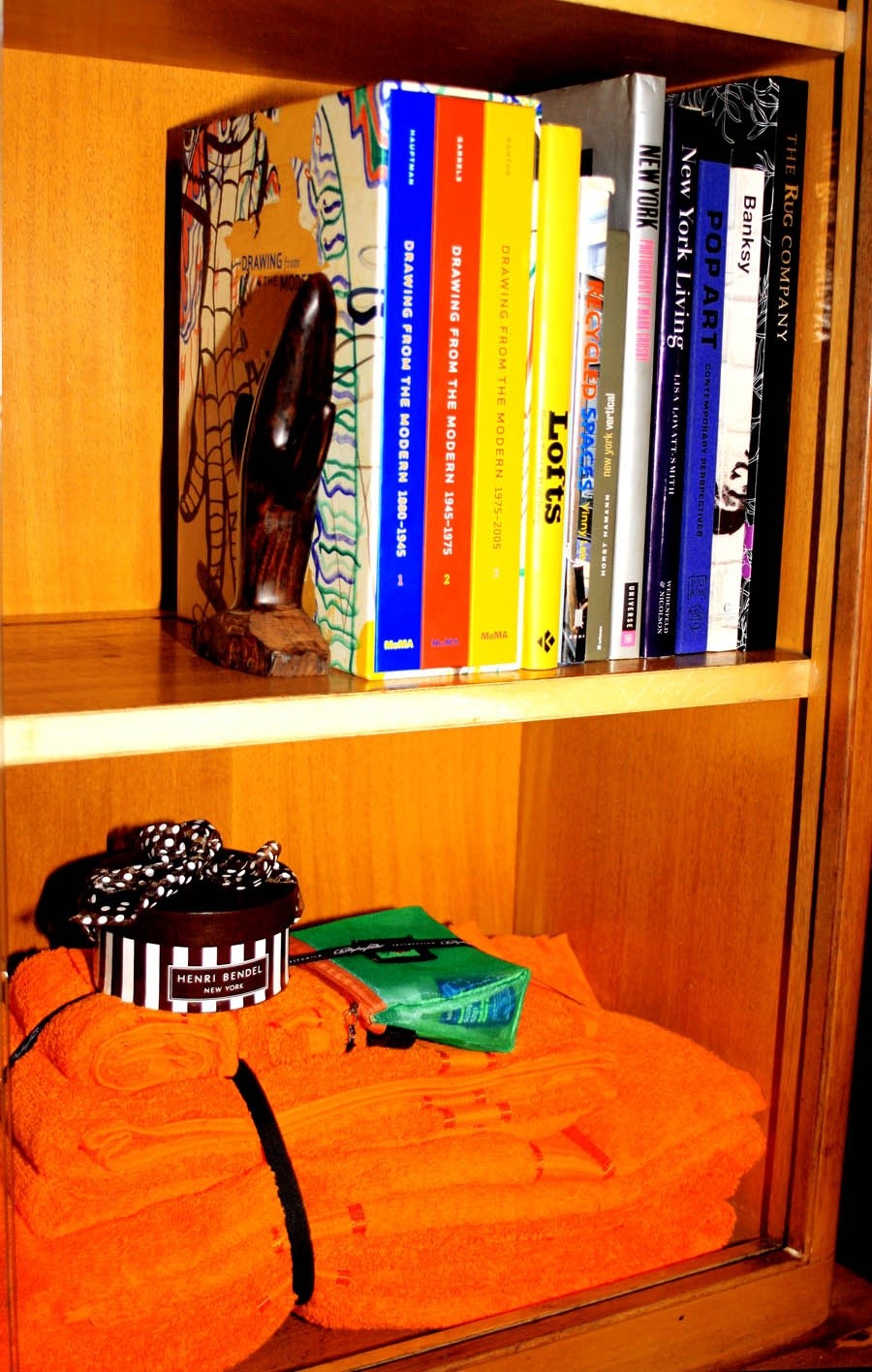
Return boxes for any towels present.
[1,914,776,1369]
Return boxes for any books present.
[174,72,810,683]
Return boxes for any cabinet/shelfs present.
[0,0,872,1372]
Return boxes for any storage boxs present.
[88,846,300,1019]
